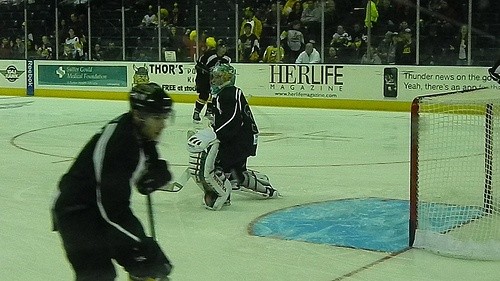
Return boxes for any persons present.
[187,64,274,209]
[52,81,176,281]
[1,0,496,64]
[193,38,228,124]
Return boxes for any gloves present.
[187,125,217,149]
[126,238,172,281]
[136,159,171,194]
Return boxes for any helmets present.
[211,63,236,94]
[217,39,228,47]
[129,82,172,114]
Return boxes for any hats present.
[161,8,169,14]
[404,27,413,33]
[391,30,398,34]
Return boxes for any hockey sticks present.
[157,167,190,192]
[146,192,157,239]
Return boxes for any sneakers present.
[204,109,214,120]
[192,108,201,123]
